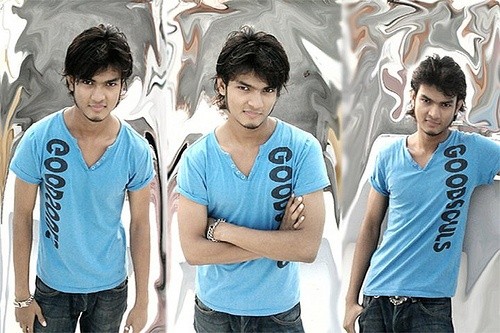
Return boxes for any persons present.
[7,23,154,333]
[343,52,500,333]
[173,27,331,332]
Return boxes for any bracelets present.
[207,217,226,243]
[13,295,34,308]
